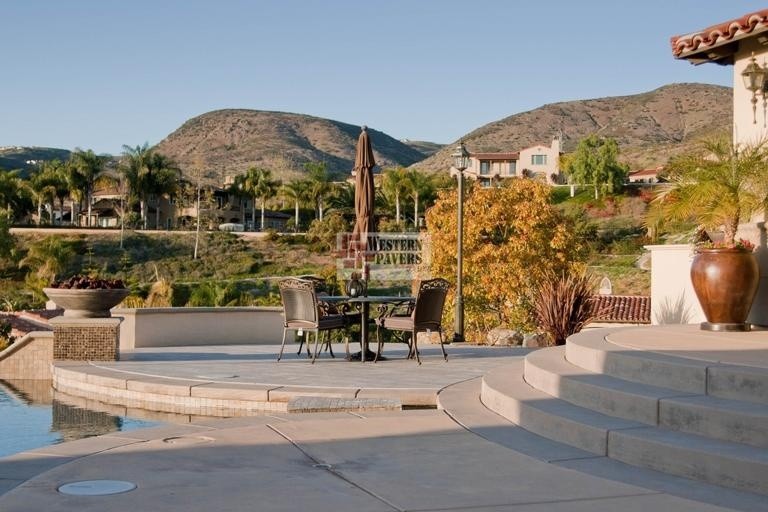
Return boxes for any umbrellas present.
[348,126,378,362]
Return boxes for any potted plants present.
[42,272,131,317]
[647,135,767,334]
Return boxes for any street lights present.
[451,135,472,343]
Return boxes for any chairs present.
[271,274,346,363]
[300,275,350,357]
[372,277,453,365]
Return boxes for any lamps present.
[741,49,766,126]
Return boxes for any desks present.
[315,294,413,361]
